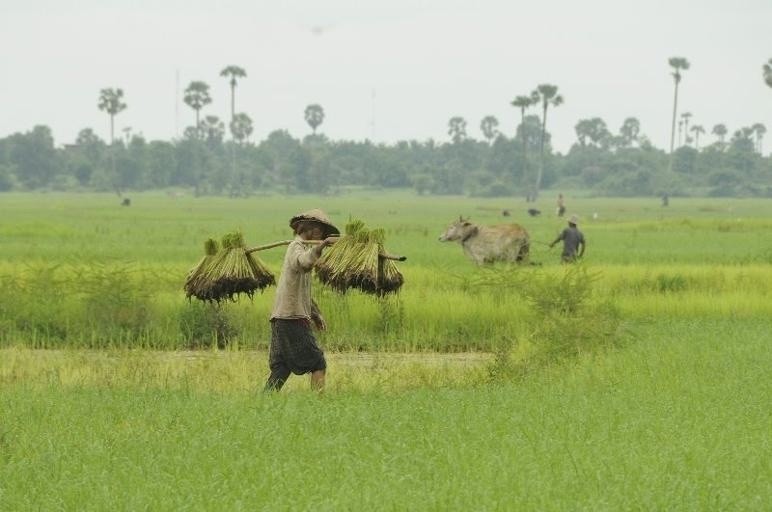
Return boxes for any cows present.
[436,215,532,269]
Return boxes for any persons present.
[548,214,586,265]
[263,208,340,394]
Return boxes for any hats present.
[289,209,340,240]
[567,216,578,224]
